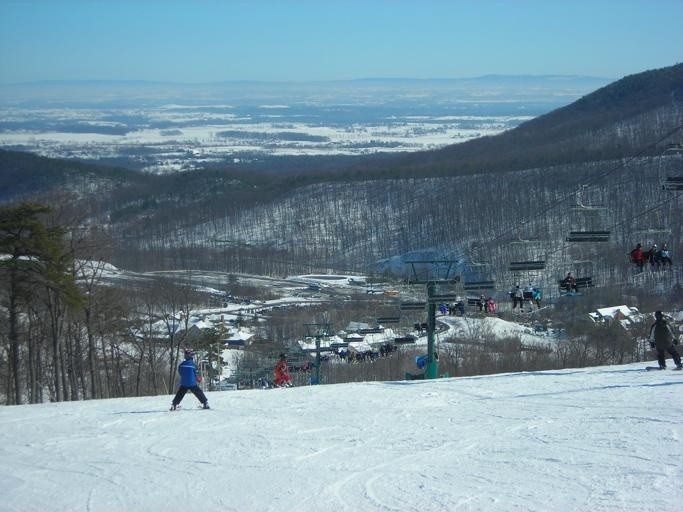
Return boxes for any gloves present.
[673,338,679,345]
[650,341,655,348]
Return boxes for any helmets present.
[184,348,195,360]
[655,311,663,320]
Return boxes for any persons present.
[437,300,467,315]
[648,310,683,370]
[512,284,526,313]
[338,342,392,364]
[563,272,578,293]
[660,244,673,267]
[648,244,661,268]
[478,294,498,312]
[170,355,210,409]
[275,352,297,388]
[630,243,645,273]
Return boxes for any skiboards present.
[513,306,549,316]
[169,404,216,411]
[645,365,683,371]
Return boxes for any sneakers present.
[677,362,682,370]
[171,402,178,409]
[203,401,209,408]
[659,362,666,368]
